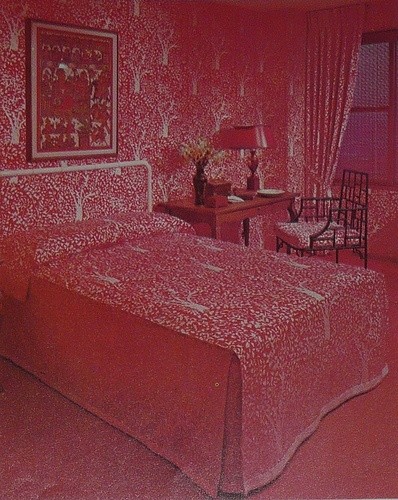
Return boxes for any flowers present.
[181,137,217,174]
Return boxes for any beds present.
[1,160,393,500]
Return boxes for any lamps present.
[230,126,277,192]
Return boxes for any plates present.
[258,189,286,197]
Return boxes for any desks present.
[158,190,302,255]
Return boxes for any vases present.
[193,163,209,206]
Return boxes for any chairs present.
[276,169,372,269]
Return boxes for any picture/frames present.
[25,22,121,161]
